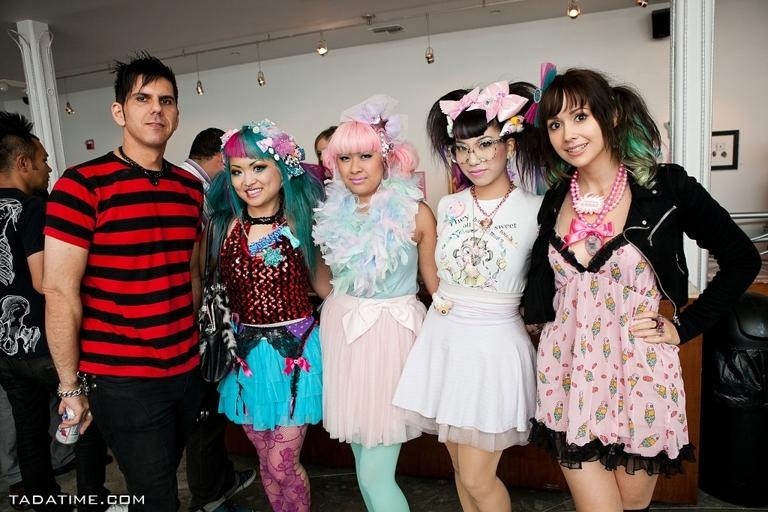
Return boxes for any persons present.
[180,125,225,188]
[313,126,337,181]
[517,63,761,512]
[314,93,438,512]
[42,55,204,512]
[205,119,326,512]
[0,111,72,512]
[382,83,543,512]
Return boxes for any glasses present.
[446,138,511,164]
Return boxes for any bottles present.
[55,407,83,445]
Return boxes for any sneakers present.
[53,451,76,476]
[199,466,256,512]
[10,482,33,511]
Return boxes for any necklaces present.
[114,145,169,187]
[564,165,628,259]
[470,180,514,233]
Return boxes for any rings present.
[652,315,666,338]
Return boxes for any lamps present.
[425,15,434,64]
[257,43,264,87]
[196,52,204,95]
[363,13,374,26]
[65,75,74,115]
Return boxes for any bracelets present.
[56,382,86,401]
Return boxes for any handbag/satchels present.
[193,216,252,384]
